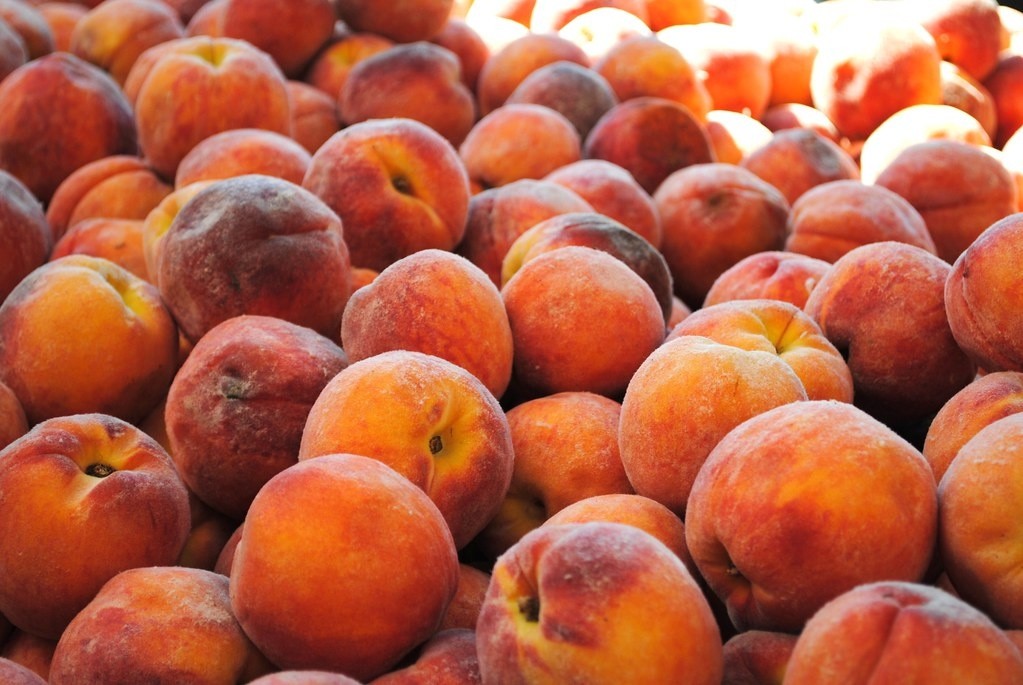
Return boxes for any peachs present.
[0,0,1023,685]
